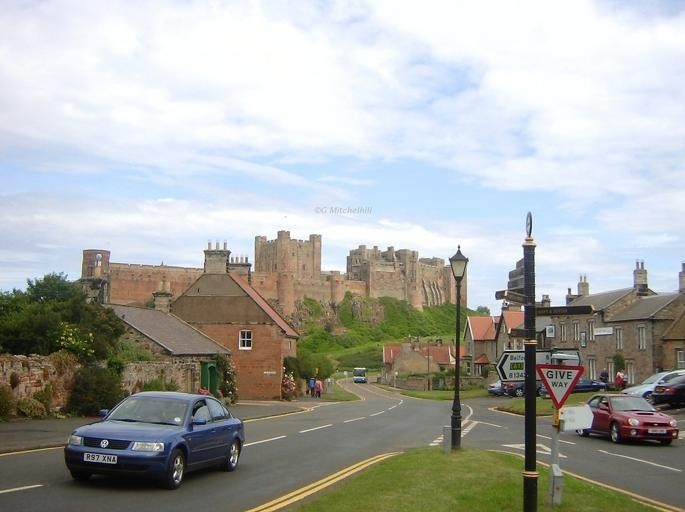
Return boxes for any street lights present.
[448,242,469,450]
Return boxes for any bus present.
[353,368,367,383]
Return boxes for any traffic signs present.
[495,350,552,381]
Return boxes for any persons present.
[197,386,212,395]
[216,385,225,402]
[622,368,628,388]
[308,377,316,398]
[315,377,324,398]
[288,371,295,387]
[616,368,624,390]
[600,368,608,392]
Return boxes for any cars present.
[487,369,685,448]
[62,389,244,491]
